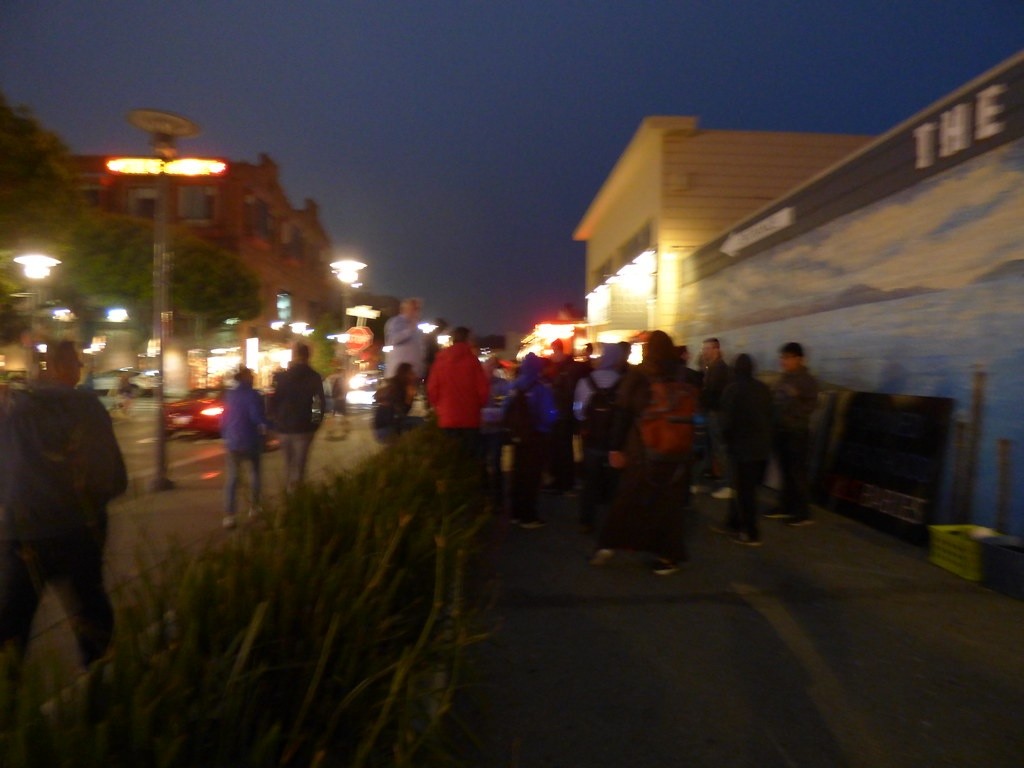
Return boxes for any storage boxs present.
[926,524,1024,596]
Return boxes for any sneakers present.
[224,515,238,530]
[248,507,264,518]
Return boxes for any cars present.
[75,368,160,400]
[164,384,275,440]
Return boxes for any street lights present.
[327,256,368,413]
[102,105,228,494]
[13,253,64,397]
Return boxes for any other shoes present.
[690,484,815,547]
[510,519,691,574]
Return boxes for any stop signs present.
[345,327,367,351]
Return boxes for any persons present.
[268,341,327,498]
[425,319,820,575]
[384,298,428,380]
[371,362,423,445]
[503,350,557,527]
[0,331,129,755]
[219,367,266,527]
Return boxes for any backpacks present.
[583,372,626,453]
[639,384,696,465]
[502,382,538,436]
